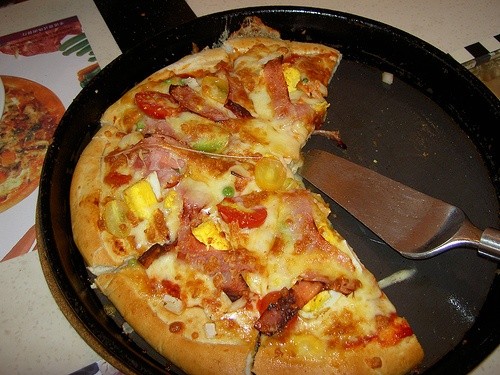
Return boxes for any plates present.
[0,74,67,217]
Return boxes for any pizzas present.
[0,75,65,212]
[68,17,424,375]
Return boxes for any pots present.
[37,6,500,375]
